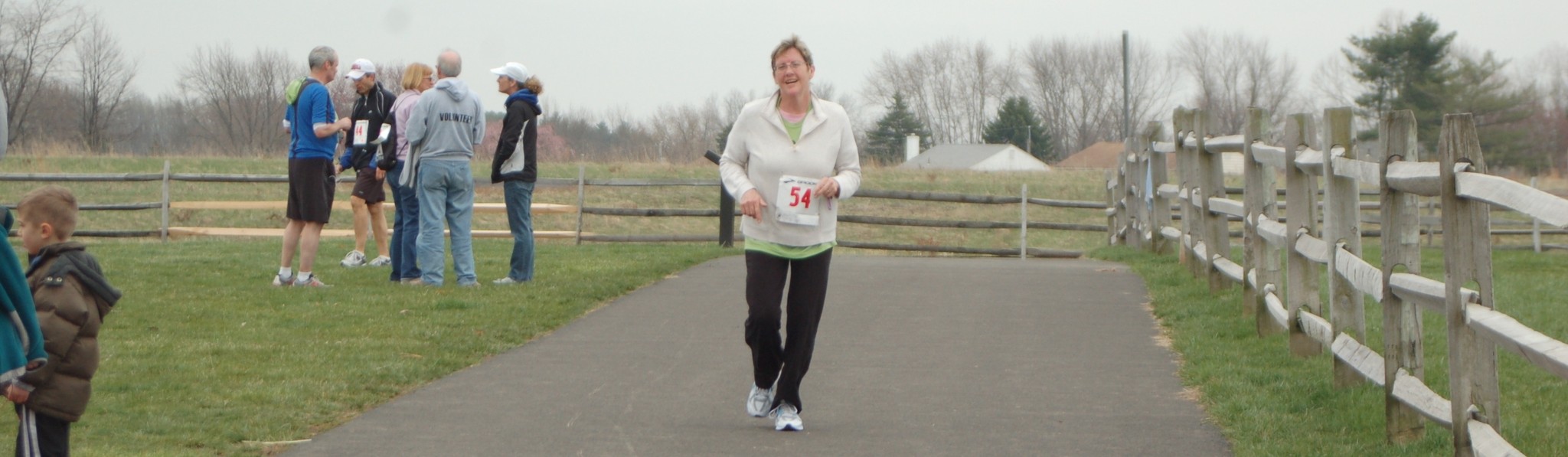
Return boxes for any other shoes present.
[401,277,416,284]
[272,271,297,287]
[369,254,391,266]
[289,273,334,288]
[411,278,436,286]
[493,276,523,285]
[341,252,366,267]
[466,280,483,287]
[390,277,400,283]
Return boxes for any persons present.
[0,185,121,457]
[719,34,863,434]
[272,45,543,287]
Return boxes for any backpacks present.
[375,93,411,170]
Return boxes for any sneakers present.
[748,378,774,417]
[769,400,804,431]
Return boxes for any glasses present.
[422,74,435,80]
[774,60,808,69]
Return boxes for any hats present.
[346,59,377,79]
[490,62,528,82]
